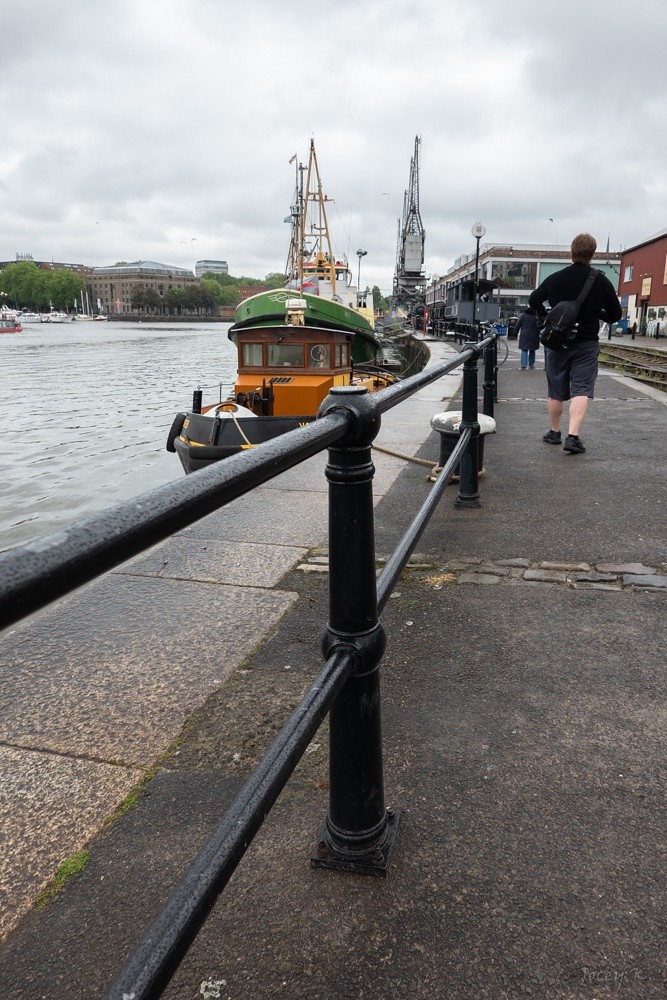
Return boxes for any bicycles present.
[487,323,508,366]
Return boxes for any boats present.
[1,290,107,333]
[164,134,401,475]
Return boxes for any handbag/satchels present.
[538,301,581,350]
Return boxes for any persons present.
[514,303,540,370]
[528,233,622,453]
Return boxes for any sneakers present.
[563,437,585,452]
[543,430,562,444]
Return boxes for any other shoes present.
[521,365,534,370]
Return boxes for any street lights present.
[470,222,485,324]
[356,249,364,291]
[432,274,438,309]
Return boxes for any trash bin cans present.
[508,317,518,340]
[618,320,629,334]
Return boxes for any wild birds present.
[382,192,390,196]
[549,218,553,222]
[191,238,196,242]
[96,222,98,224]
